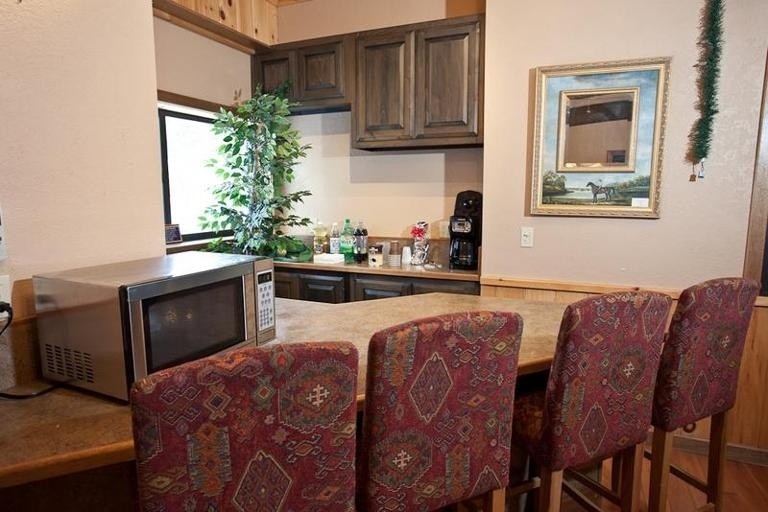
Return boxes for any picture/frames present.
[530,57,671,219]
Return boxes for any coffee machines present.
[446,190,483,269]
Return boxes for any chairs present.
[358,311,524,512]
[610,276,761,512]
[129,340,359,511]
[514,291,672,512]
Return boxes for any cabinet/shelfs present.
[351,12,484,147]
[273,270,342,304]
[354,277,476,302]
[251,32,355,116]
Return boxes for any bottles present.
[311,217,368,263]
[400,246,412,264]
[388,240,401,266]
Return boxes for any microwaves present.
[31,246,277,408]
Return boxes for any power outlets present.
[0,293,11,319]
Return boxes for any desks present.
[0,289,624,496]
